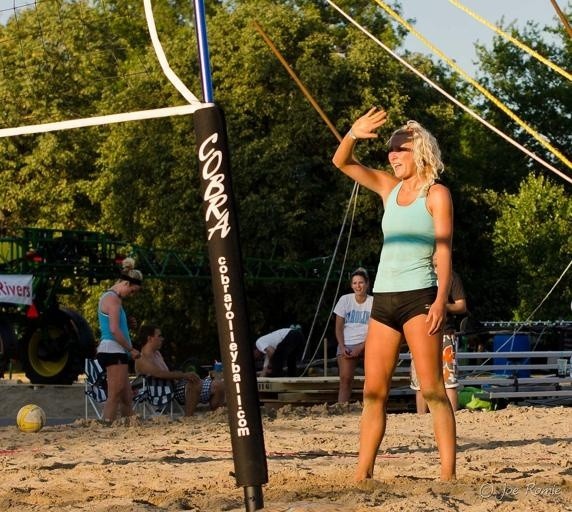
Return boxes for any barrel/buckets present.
[493,332,532,378]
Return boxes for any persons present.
[332,267,374,403]
[133,323,227,416]
[409,250,467,415]
[332,107,455,482]
[94,257,144,423]
[249,326,302,376]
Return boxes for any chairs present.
[85,357,185,420]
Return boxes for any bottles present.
[214,362,223,381]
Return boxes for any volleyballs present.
[17,404,46,433]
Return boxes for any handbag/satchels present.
[459,387,507,411]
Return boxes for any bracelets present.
[348,128,357,141]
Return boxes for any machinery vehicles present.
[0,224,376,388]
